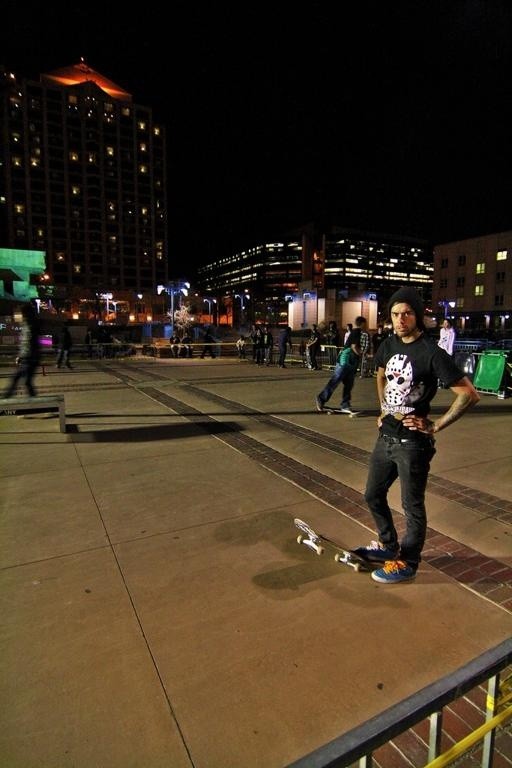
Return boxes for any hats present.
[387,285,423,328]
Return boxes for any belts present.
[380,432,433,444]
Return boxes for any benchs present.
[155,341,193,357]
[0,395,65,433]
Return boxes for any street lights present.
[157,281,190,326]
[285,291,310,329]
[203,296,218,324]
[100,290,113,315]
[234,294,250,325]
[438,299,449,320]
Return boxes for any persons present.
[199,331,219,359]
[348,285,480,586]
[180,331,195,360]
[370,323,387,355]
[356,326,369,374]
[2,301,42,421]
[343,322,352,346]
[169,331,182,357]
[306,323,321,372]
[316,316,367,415]
[436,313,457,389]
[236,335,246,359]
[249,324,293,369]
[84,328,94,359]
[55,320,76,370]
[322,321,340,368]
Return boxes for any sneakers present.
[315,395,324,412]
[340,406,361,415]
[354,540,400,563]
[369,558,418,584]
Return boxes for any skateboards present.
[294,518,374,572]
[361,354,367,378]
[323,405,363,418]
